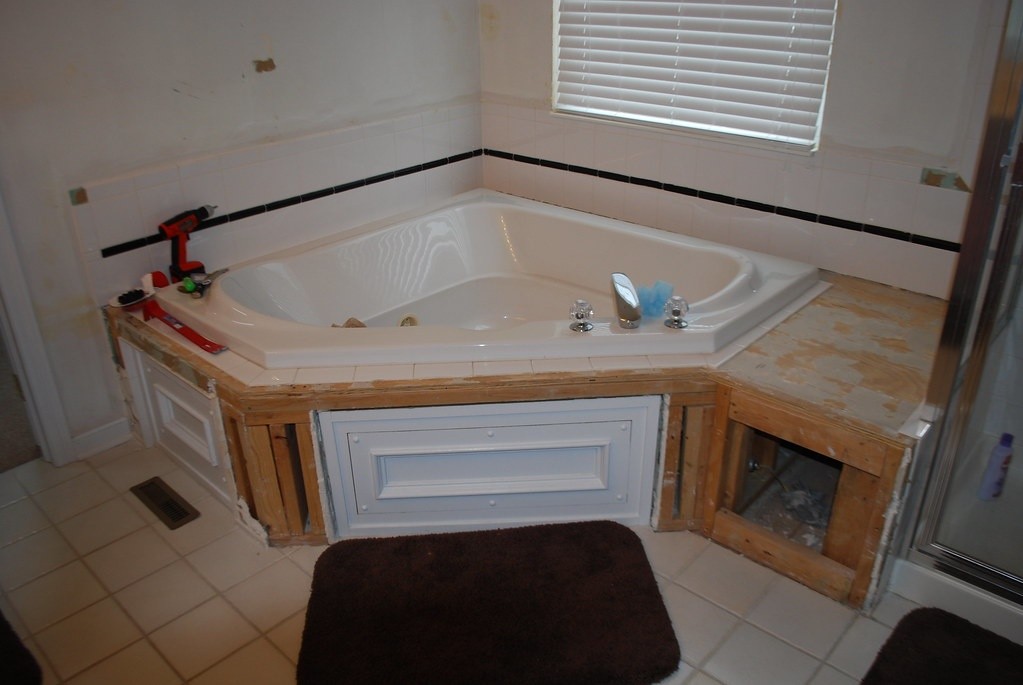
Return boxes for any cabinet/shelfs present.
[100,306,243,521]
[267,377,717,548]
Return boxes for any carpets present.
[293,520,680,685]
[858,606,1023,685]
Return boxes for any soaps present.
[139,272,157,296]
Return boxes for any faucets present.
[609,269,644,330]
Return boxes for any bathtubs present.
[105,184,824,555]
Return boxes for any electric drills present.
[157,204,219,284]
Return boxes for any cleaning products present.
[974,429,1016,507]
